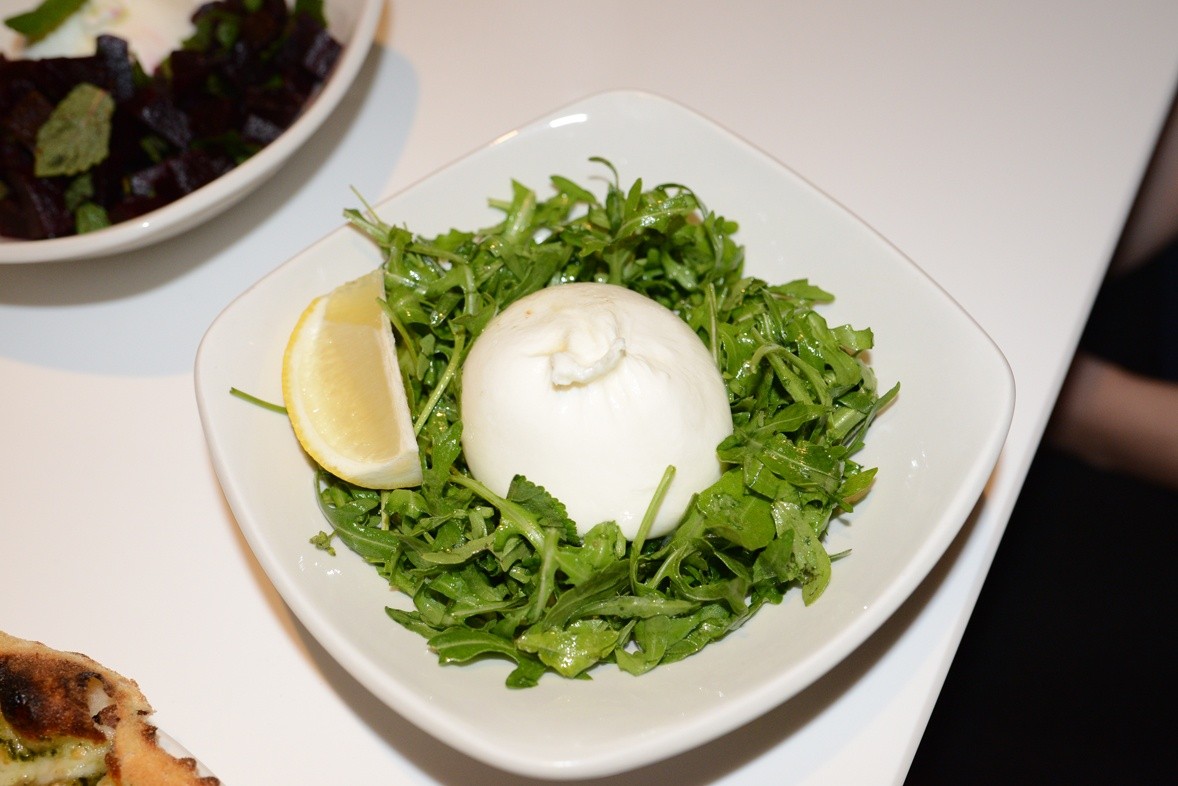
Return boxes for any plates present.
[193,87,1016,777]
[0,0,385,264]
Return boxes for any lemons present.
[276,246,420,492]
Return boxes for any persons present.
[1033,106,1178,506]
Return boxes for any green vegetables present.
[289,146,902,691]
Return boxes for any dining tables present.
[1,0,1177,785]
